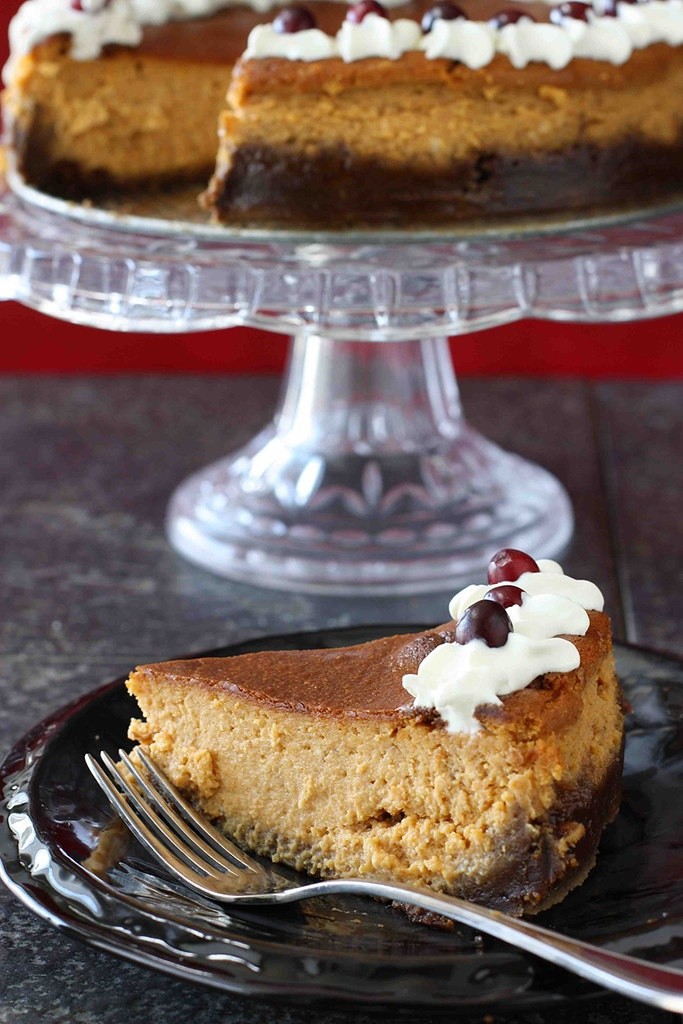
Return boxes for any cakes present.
[108,549,626,928]
[0,0,683,230]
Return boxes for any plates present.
[1,622,683,1012]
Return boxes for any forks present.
[84,745,683,1015]
